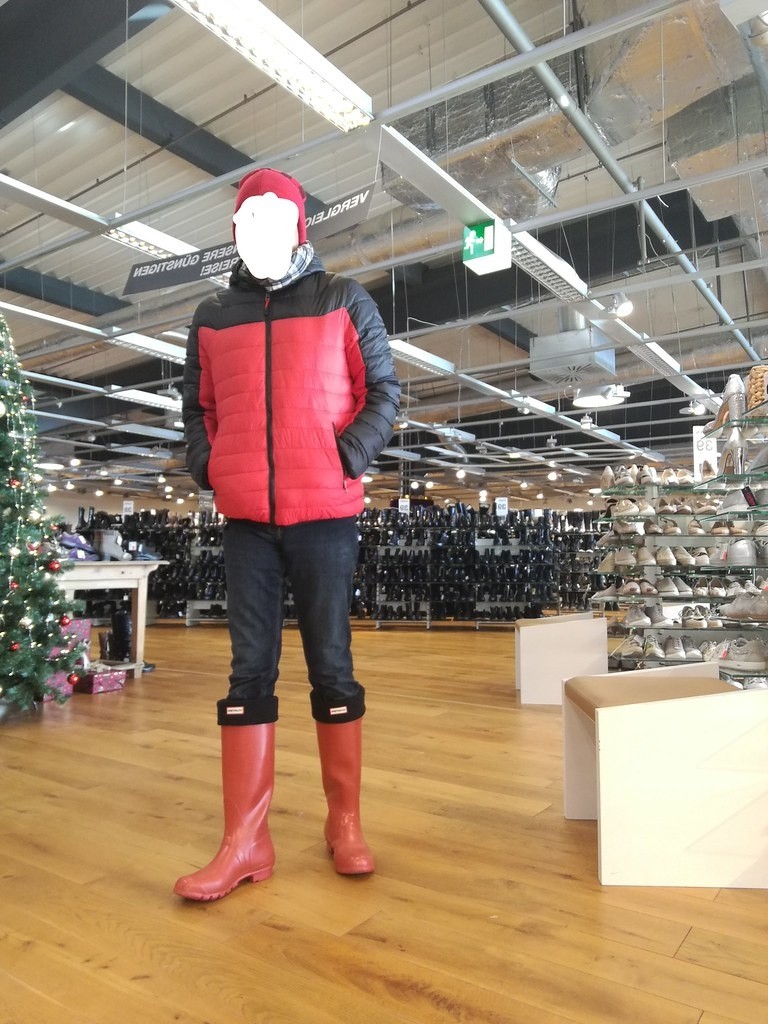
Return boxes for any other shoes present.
[61,532,94,552]
[63,548,100,561]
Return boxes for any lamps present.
[166,383,181,401]
[99,466,109,476]
[105,0,727,490]
[48,484,56,493]
[536,490,544,499]
[456,468,466,480]
[156,475,166,484]
[81,429,96,442]
[114,477,123,485]
[479,489,488,497]
[520,479,528,488]
[187,489,195,498]
[688,401,707,418]
[96,488,103,497]
[605,291,634,319]
[410,480,420,490]
[64,481,75,491]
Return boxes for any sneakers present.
[591,438,768,688]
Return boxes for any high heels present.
[716,428,744,482]
[703,374,746,432]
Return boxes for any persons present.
[176,169,401,900]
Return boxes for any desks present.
[42,560,171,679]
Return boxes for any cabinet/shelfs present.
[75,397,768,691]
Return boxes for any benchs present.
[514,612,608,705]
[561,662,768,890]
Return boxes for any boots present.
[99,632,108,660]
[175,697,277,901]
[76,505,622,623]
[111,613,156,673]
[310,684,378,875]
[106,631,114,660]
[93,528,132,562]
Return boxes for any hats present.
[231,167,307,247]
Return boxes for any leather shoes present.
[135,550,163,561]
